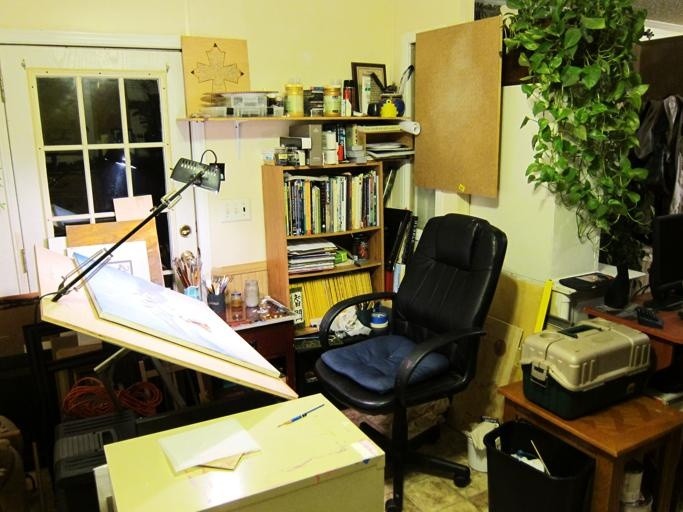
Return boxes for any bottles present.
[285,86,303,116]
[232,293,242,307]
[323,88,340,115]
[245,280,258,306]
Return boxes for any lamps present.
[52,151,218,302]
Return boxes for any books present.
[288,272,379,336]
[287,238,337,275]
[368,141,416,160]
[384,209,418,294]
[283,170,380,236]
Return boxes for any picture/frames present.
[350,59,387,114]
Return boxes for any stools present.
[497,379,683,512]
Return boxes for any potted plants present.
[500,0,655,252]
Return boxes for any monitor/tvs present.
[649,214,683,305]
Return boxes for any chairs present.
[315,212,509,512]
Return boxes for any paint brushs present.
[205,274,230,296]
[172,256,203,288]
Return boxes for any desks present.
[37,296,298,441]
[583,290,683,372]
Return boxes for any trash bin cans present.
[467,422,501,472]
[483,418,597,512]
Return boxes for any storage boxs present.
[521,315,652,420]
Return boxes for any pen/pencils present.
[278,404,323,426]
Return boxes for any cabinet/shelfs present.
[261,160,386,339]
